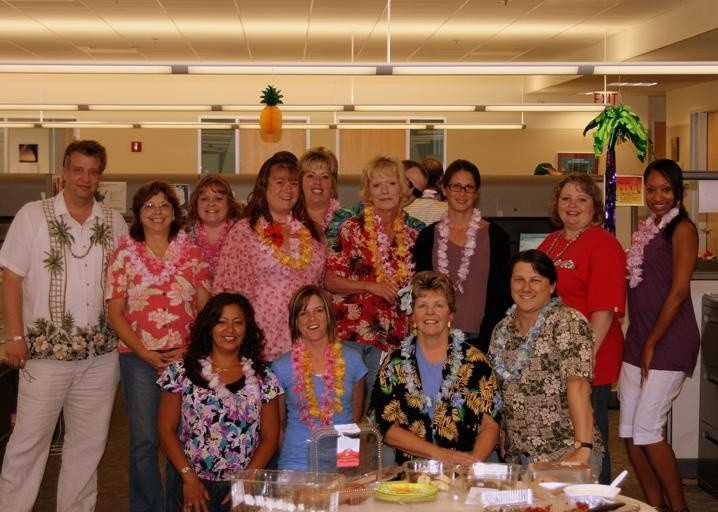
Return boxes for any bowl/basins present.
[562,483,620,500]
[370,482,438,501]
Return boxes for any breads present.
[291,482,368,508]
[530,476,562,500]
[408,469,451,492]
[467,478,514,491]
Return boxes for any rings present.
[455,464,461,469]
[189,502,192,506]
[20,359,26,363]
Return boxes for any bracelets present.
[178,465,191,475]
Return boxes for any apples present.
[703,250,713,257]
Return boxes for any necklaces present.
[546,225,592,262]
[58,212,99,258]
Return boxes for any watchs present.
[574,440,592,449]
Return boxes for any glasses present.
[143,201,171,213]
[406,178,424,198]
[446,183,475,194]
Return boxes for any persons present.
[618,157,701,512]
[271,284,370,495]
[156,293,285,512]
[486,250,606,482]
[3,335,23,345]
[371,269,505,480]
[108,179,211,511]
[0,142,131,512]
[186,144,511,417]
[536,171,628,483]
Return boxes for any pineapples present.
[260,84,285,144]
